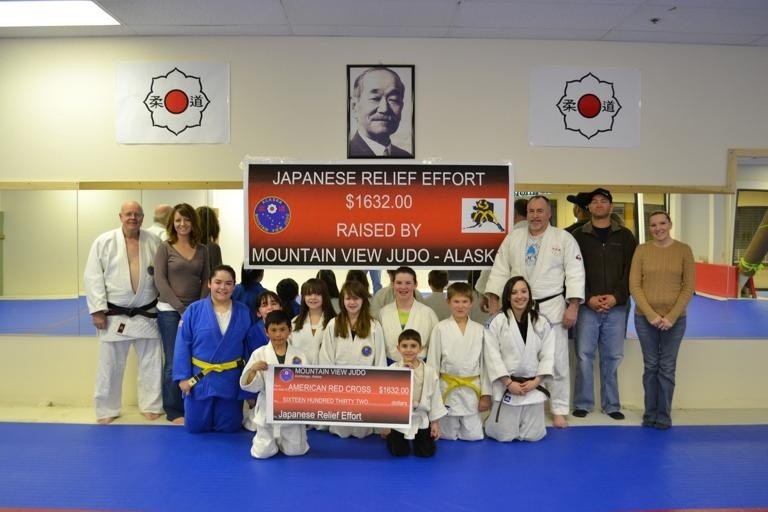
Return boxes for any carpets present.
[1,300,108,336]
[1,422,768,511]
[625,289,768,338]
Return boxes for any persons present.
[379,267,440,364]
[246,292,282,360]
[233,261,268,303]
[317,269,339,313]
[84,200,164,423]
[197,206,220,246]
[239,310,310,459]
[276,279,301,315]
[469,270,492,323]
[350,68,413,156]
[175,266,257,432]
[630,210,695,430]
[146,205,172,240]
[484,196,586,428]
[484,276,555,442]
[563,192,591,233]
[153,204,209,424]
[514,198,530,222]
[346,270,371,302]
[571,188,637,419]
[320,282,388,439]
[290,279,337,367]
[424,270,451,321]
[426,282,493,441]
[371,270,423,317]
[388,328,448,458]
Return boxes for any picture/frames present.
[346,64,414,160]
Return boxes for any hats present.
[588,188,612,202]
[567,192,589,209]
